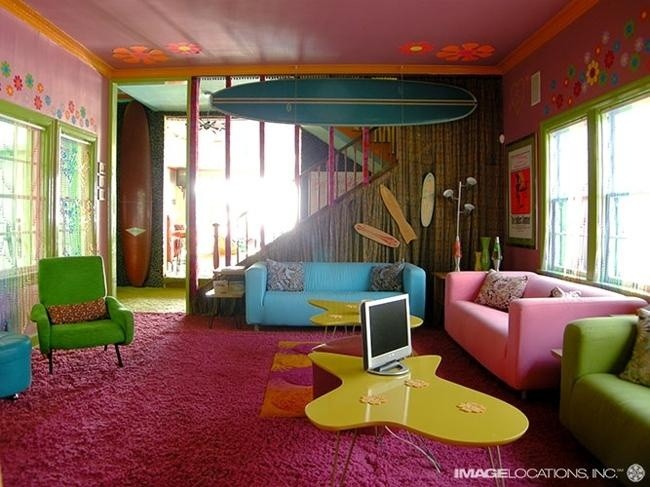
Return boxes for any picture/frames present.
[99,175,106,187]
[99,161,107,175]
[99,189,107,200]
[504,133,536,246]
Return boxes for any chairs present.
[29,255,134,375]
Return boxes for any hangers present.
[444,177,476,269]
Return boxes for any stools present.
[0,330,31,398]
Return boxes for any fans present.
[187,111,220,134]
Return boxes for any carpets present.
[115,287,186,313]
[0,311,614,487]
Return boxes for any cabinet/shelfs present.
[434,272,448,308]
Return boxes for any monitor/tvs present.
[360,293,413,376]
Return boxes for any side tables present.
[204,290,244,329]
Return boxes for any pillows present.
[552,287,580,298]
[621,308,650,387]
[265,258,305,291]
[367,258,404,290]
[475,268,527,310]
[48,297,106,323]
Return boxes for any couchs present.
[445,269,648,400]
[244,261,426,331]
[562,316,650,464]
